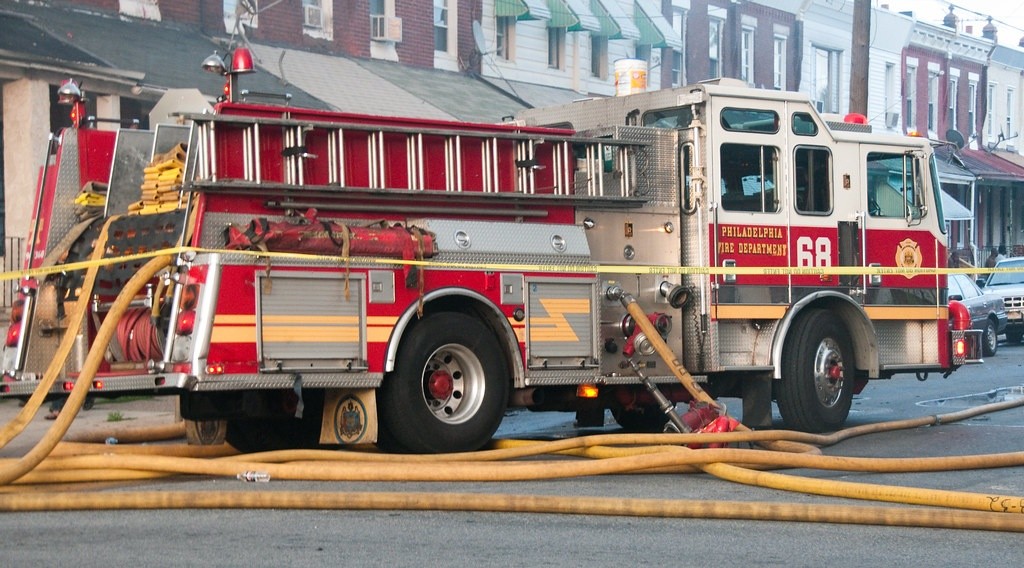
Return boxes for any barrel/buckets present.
[614,59,648,96]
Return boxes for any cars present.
[948,274,1009,356]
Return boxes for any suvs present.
[975,257,1024,343]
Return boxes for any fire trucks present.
[0,47,989,451]
